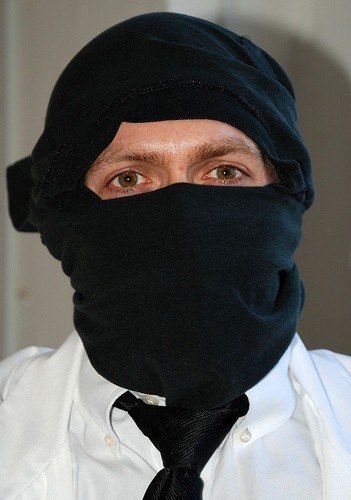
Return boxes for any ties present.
[116,390,250,500]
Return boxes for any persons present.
[0,12,349,499]
